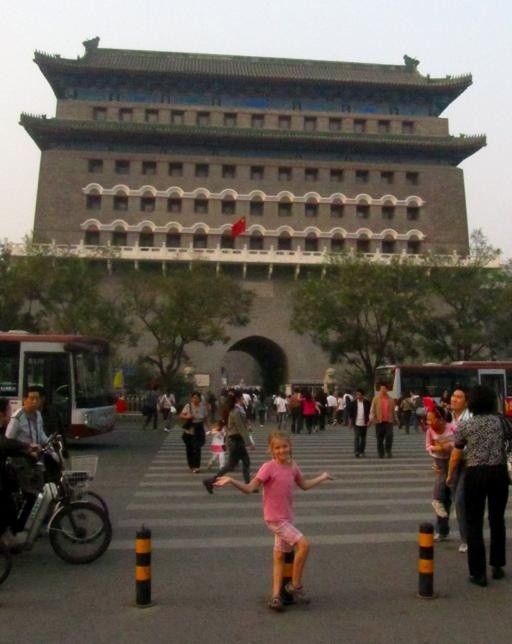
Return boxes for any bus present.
[0,328,119,439]
[450,360,512,419]
[372,362,453,422]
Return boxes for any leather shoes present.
[203,479,213,493]
[470,575,487,585]
[493,565,504,578]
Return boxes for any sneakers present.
[286,583,310,604]
[269,597,285,610]
[453,506,458,519]
[164,428,170,432]
[459,543,468,552]
[433,531,449,541]
[431,499,448,517]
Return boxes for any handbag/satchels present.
[499,416,512,484]
[164,394,177,414]
[179,403,193,429]
[142,390,152,415]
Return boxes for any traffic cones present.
[417,519,439,599]
[275,535,304,607]
[133,525,154,607]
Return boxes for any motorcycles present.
[0,431,113,566]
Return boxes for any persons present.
[327,390,335,428]
[408,389,419,435]
[217,386,233,430]
[397,392,405,429]
[417,387,434,413]
[235,387,292,429]
[2,397,41,546]
[298,388,320,435]
[203,393,258,494]
[335,391,346,426]
[439,388,452,417]
[289,387,304,434]
[426,404,459,518]
[348,387,371,459]
[368,382,399,460]
[209,392,219,417]
[179,390,207,472]
[141,383,160,429]
[399,393,413,435]
[445,384,511,586]
[314,382,329,434]
[212,433,336,612]
[342,386,352,428]
[206,420,226,473]
[159,387,176,433]
[7,385,59,483]
[429,386,470,552]
